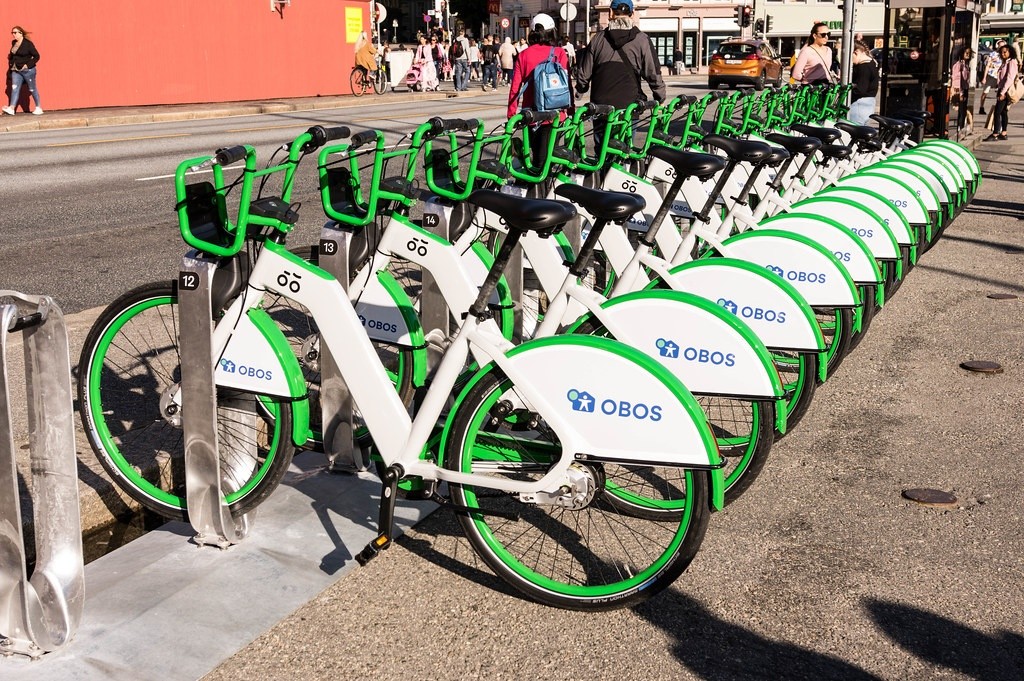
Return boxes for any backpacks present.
[453,38,464,57]
[483,45,494,63]
[515,47,571,112]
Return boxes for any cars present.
[870,47,916,80]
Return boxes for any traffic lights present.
[734,5,743,26]
[766,15,773,33]
[758,20,764,32]
[743,6,750,28]
[589,6,598,27]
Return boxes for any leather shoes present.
[982,134,999,142]
[998,134,1008,140]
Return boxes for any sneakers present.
[2,107,14,115]
[32,107,43,115]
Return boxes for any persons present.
[574,0,666,154]
[951,48,976,130]
[673,47,683,75]
[2,26,43,116]
[354,26,586,92]
[848,32,879,126]
[979,36,1023,142]
[790,23,842,88]
[508,13,575,166]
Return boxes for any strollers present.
[405,61,424,92]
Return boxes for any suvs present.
[708,36,782,90]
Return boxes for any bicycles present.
[719,90,954,238]
[690,91,942,249]
[314,121,784,520]
[743,87,982,222]
[425,117,830,445]
[75,123,723,610]
[656,94,933,266]
[587,101,903,306]
[559,104,886,354]
[619,98,916,286]
[349,54,387,97]
[497,110,865,384]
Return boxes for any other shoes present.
[979,107,987,115]
[461,88,469,91]
[482,84,487,91]
[492,88,498,91]
[502,80,510,86]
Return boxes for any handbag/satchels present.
[984,105,995,130]
[1007,75,1024,105]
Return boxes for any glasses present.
[818,32,831,38]
[11,32,20,34]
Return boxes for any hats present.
[610,0,633,11]
[530,14,555,31]
